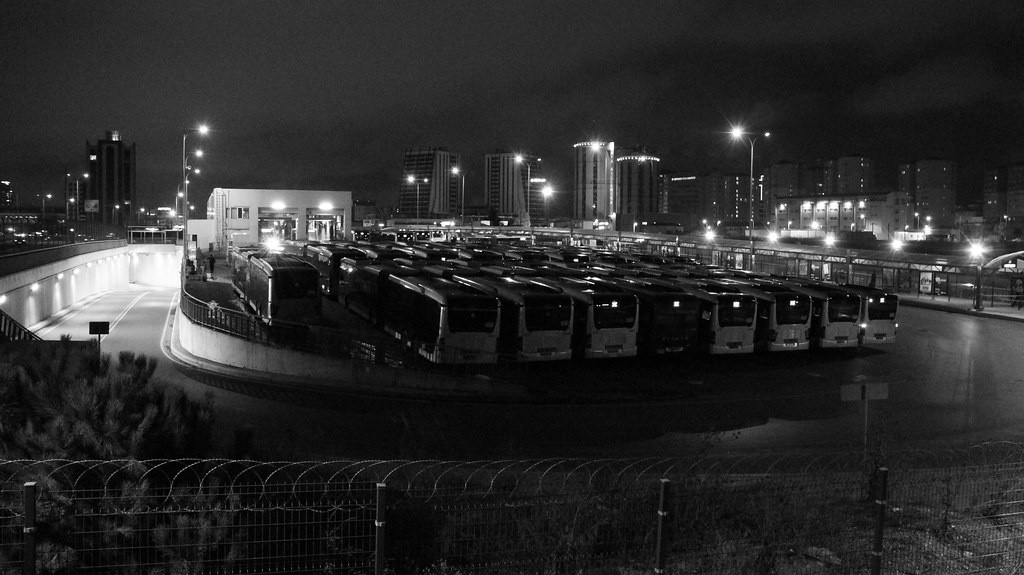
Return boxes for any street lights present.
[185,150,204,254]
[453,168,464,225]
[928,216,931,227]
[972,244,983,308]
[915,213,920,230]
[735,129,771,248]
[516,156,541,226]
[594,144,608,223]
[410,177,428,224]
[182,128,205,277]
[66,173,88,220]
[36,194,52,213]
[175,181,189,219]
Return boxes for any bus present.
[662,275,758,357]
[451,274,575,368]
[306,239,770,323]
[624,274,700,360]
[381,273,502,367]
[515,274,640,360]
[232,245,322,328]
[751,278,863,350]
[786,275,900,348]
[709,277,813,352]
